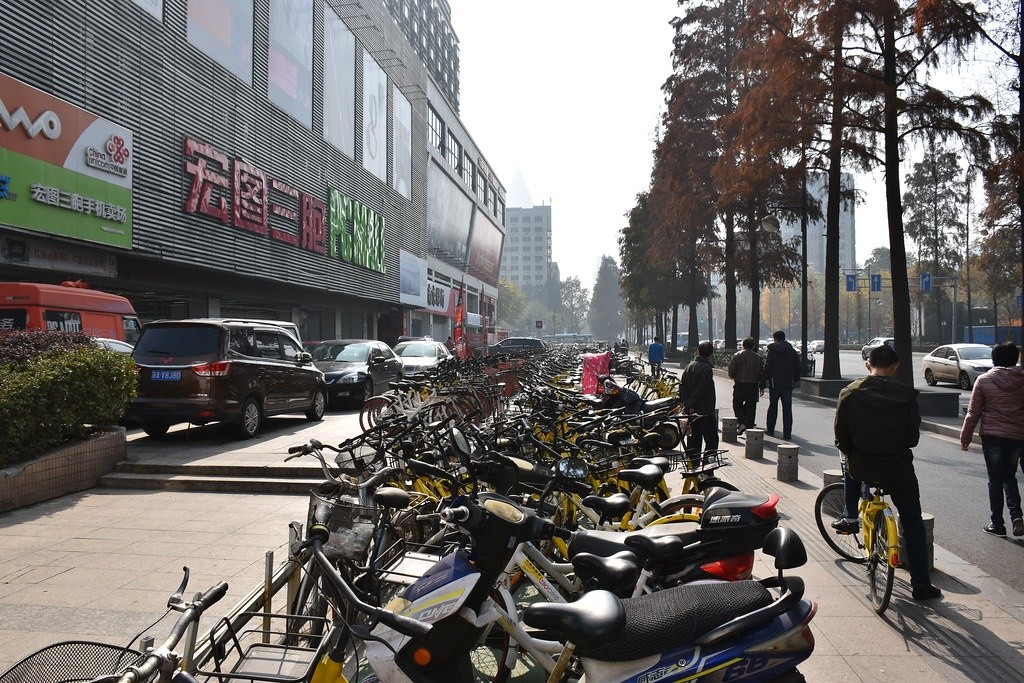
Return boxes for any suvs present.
[124,317,328,437]
[473,336,546,359]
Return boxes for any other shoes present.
[832,517,859,534]
[737,424,746,435]
[783,435,792,439]
[912,584,942,600]
[764,429,774,437]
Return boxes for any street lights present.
[528,308,531,336]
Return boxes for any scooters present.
[348,427,818,683]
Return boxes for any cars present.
[300,339,406,410]
[861,337,895,359]
[385,341,455,388]
[697,337,825,359]
[922,343,996,391]
[92,337,135,356]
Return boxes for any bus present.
[542,333,596,343]
[676,332,702,351]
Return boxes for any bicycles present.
[814,449,902,614]
[283,345,737,646]
[0,566,630,683]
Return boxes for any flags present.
[453,281,472,358]
[481,288,488,357]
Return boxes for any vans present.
[203,318,303,351]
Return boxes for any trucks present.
[0,280,143,345]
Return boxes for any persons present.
[446,337,455,355]
[764,331,802,439]
[614,342,621,353]
[558,344,563,352]
[728,336,766,435]
[573,343,578,350]
[648,336,664,379]
[831,345,941,600]
[619,339,626,353]
[543,340,548,348]
[960,342,1024,538]
[625,340,629,355]
[679,342,721,482]
[587,344,608,350]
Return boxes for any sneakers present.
[983,522,1007,537]
[1013,517,1024,536]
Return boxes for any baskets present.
[586,448,728,476]
[0,442,383,683]
